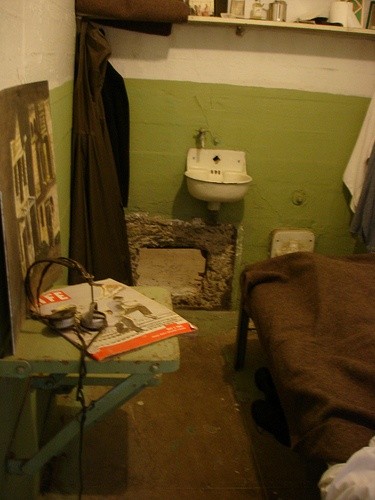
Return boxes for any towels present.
[341,93,375,244]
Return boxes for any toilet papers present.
[327,1,361,28]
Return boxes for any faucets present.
[196,128,205,148]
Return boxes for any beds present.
[233,252,375,500]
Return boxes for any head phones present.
[23,254,108,331]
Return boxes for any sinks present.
[183,147,253,203]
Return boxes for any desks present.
[0,285,181,500]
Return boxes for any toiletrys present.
[251,2,264,19]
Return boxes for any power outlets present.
[268,228,315,259]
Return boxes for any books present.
[25,275,199,363]
